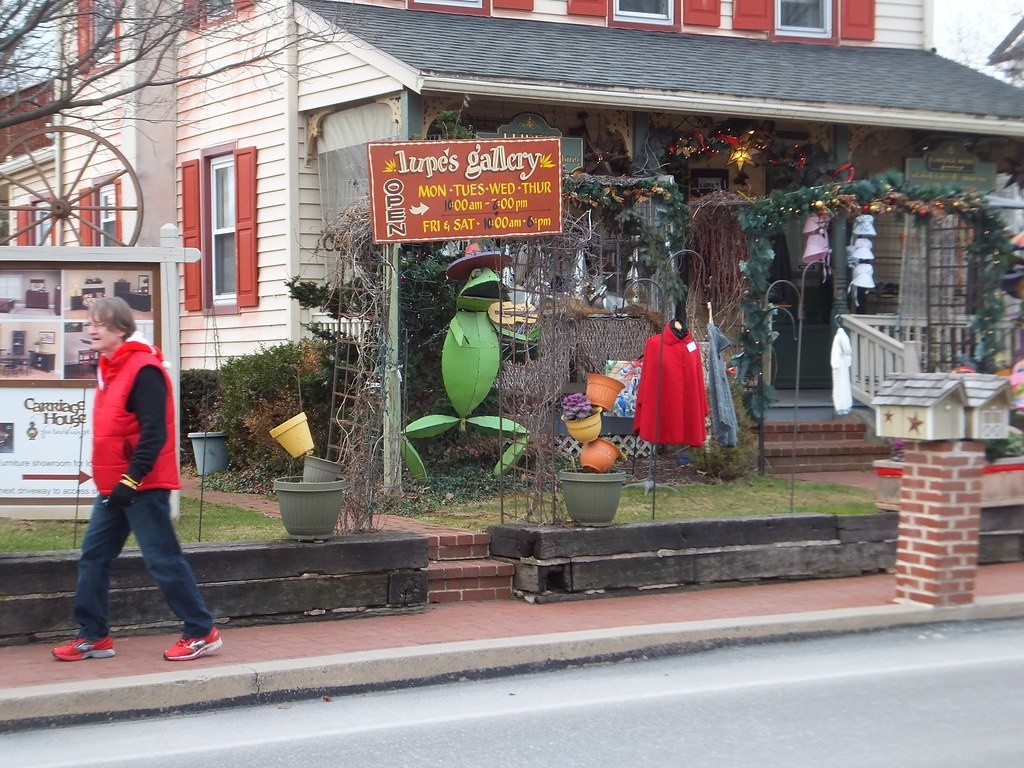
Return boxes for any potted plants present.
[187,397,234,475]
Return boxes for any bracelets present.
[120,473,139,490]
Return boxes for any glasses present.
[84,322,110,329]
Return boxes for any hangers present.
[706,302,715,326]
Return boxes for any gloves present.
[102,473,142,511]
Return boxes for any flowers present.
[561,393,594,421]
[604,432,633,473]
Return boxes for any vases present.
[561,406,602,443]
[586,372,626,412]
[274,476,347,541]
[304,455,343,482]
[580,437,621,474]
[269,411,315,458]
[560,468,626,527]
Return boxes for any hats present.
[848,264,875,294]
[852,215,877,236]
[852,237,875,259]
[802,214,825,233]
[802,234,829,263]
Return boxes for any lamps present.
[726,130,756,173]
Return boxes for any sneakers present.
[164,625,223,661]
[52,635,115,660]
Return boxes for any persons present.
[52,295,223,663]
